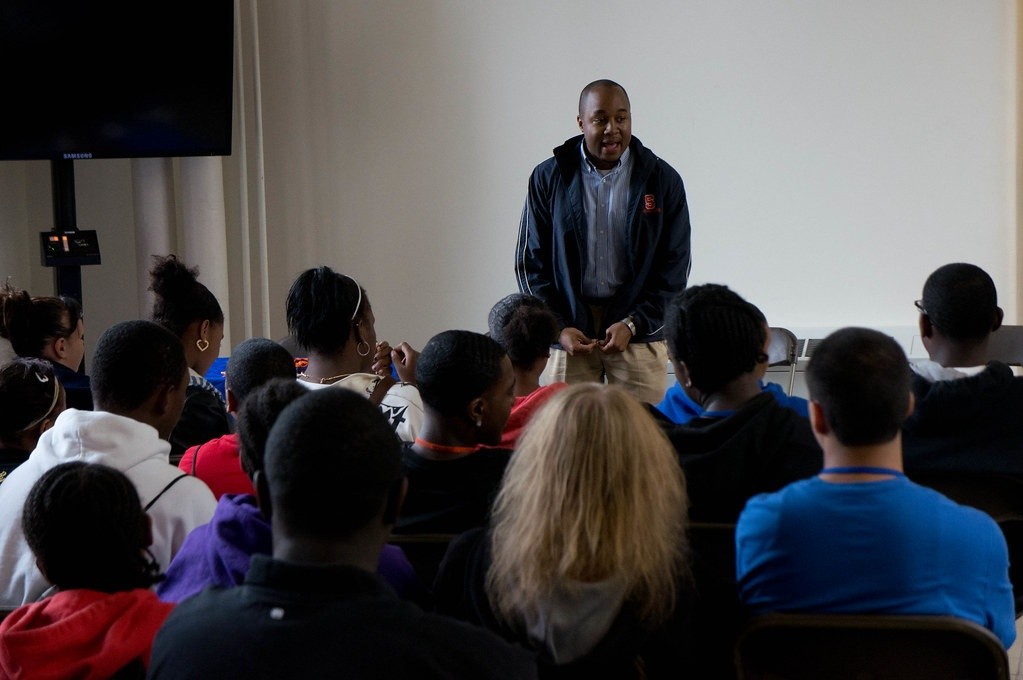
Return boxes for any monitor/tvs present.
[0,0,235,159]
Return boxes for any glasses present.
[915,299,932,338]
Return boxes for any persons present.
[515,80,692,402]
[457,379,739,680]
[286,267,424,447]
[146,388,539,680]
[178,338,295,501]
[905,264,1023,619]
[488,294,569,449]
[734,326,1016,653]
[647,283,821,526]
[1,284,291,680]
[152,259,224,405]
[397,331,517,535]
[161,379,416,604]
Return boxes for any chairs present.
[168,325,1023,680]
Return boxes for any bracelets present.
[621,318,635,336]
